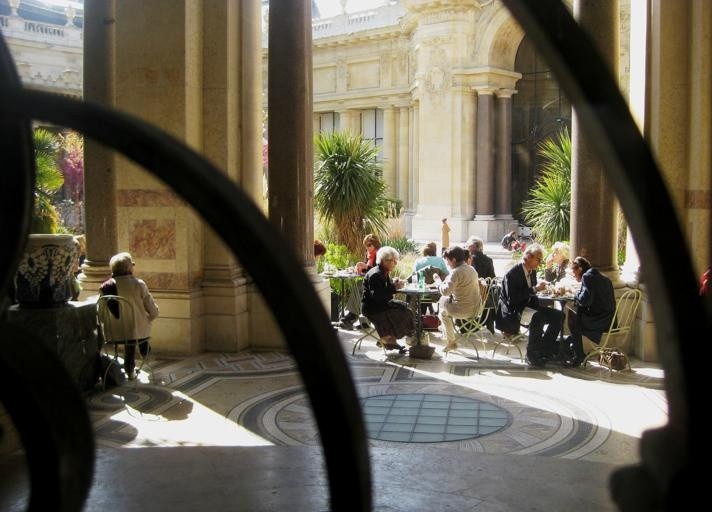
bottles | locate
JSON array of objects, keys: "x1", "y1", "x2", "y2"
[
  {"x1": 419, "y1": 272, "x2": 424, "y2": 288},
  {"x1": 412, "y1": 272, "x2": 417, "y2": 285}
]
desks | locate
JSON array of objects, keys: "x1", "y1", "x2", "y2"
[
  {"x1": 534, "y1": 286, "x2": 578, "y2": 367},
  {"x1": 322, "y1": 271, "x2": 363, "y2": 326},
  {"x1": 392, "y1": 284, "x2": 441, "y2": 361}
]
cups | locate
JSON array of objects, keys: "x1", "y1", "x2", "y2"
[{"x1": 560, "y1": 286, "x2": 565, "y2": 296}]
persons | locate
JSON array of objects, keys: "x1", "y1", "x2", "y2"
[
  {"x1": 97, "y1": 250, "x2": 159, "y2": 373},
  {"x1": 558, "y1": 254, "x2": 620, "y2": 370},
  {"x1": 312, "y1": 215, "x2": 571, "y2": 366}
]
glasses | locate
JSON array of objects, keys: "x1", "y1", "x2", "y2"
[{"x1": 367, "y1": 243, "x2": 371, "y2": 248}]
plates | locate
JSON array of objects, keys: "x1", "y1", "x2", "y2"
[
  {"x1": 430, "y1": 287, "x2": 436, "y2": 289},
  {"x1": 324, "y1": 273, "x2": 334, "y2": 275}
]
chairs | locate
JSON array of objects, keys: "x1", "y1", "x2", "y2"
[
  {"x1": 579, "y1": 289, "x2": 642, "y2": 376},
  {"x1": 445, "y1": 276, "x2": 529, "y2": 363},
  {"x1": 95, "y1": 295, "x2": 153, "y2": 392},
  {"x1": 349, "y1": 276, "x2": 388, "y2": 356}
]
